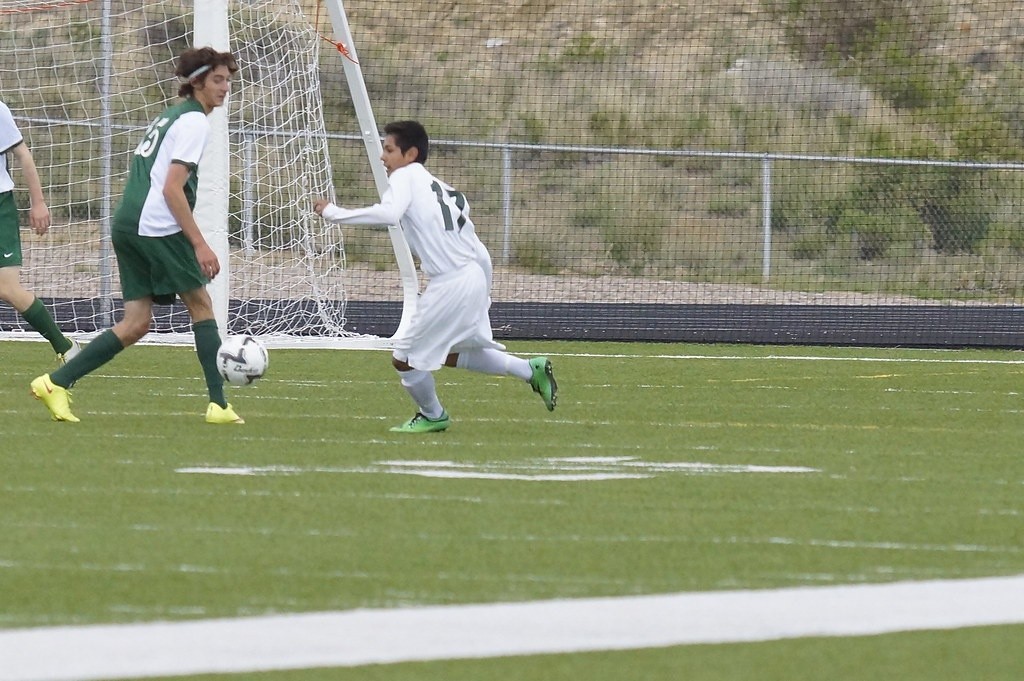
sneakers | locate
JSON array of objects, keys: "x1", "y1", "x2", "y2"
[
  {"x1": 205, "y1": 401, "x2": 245, "y2": 424},
  {"x1": 57, "y1": 336, "x2": 82, "y2": 387},
  {"x1": 526, "y1": 356, "x2": 558, "y2": 411},
  {"x1": 29, "y1": 372, "x2": 80, "y2": 423},
  {"x1": 388, "y1": 406, "x2": 450, "y2": 434}
]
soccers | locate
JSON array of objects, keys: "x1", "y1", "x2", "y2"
[{"x1": 215, "y1": 334, "x2": 270, "y2": 387}]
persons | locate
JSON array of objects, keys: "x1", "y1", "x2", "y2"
[
  {"x1": 31, "y1": 48, "x2": 245, "y2": 424},
  {"x1": 313, "y1": 120, "x2": 558, "y2": 433},
  {"x1": 0, "y1": 102, "x2": 81, "y2": 365}
]
